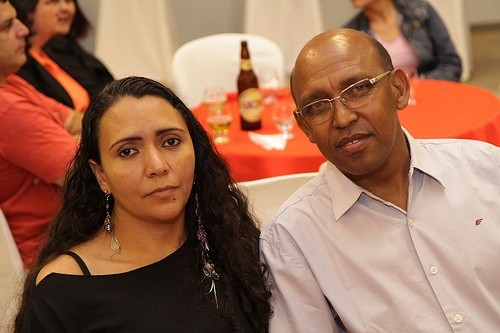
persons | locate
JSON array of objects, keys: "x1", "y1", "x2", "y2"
[
  {"x1": 259, "y1": 28, "x2": 500, "y2": 333},
  {"x1": 8, "y1": 0, "x2": 115, "y2": 111},
  {"x1": 11, "y1": 76, "x2": 275, "y2": 333},
  {"x1": 340, "y1": 0, "x2": 463, "y2": 82},
  {"x1": 0, "y1": 0, "x2": 87, "y2": 269}
]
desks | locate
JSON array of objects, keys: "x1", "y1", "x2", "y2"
[{"x1": 190, "y1": 78, "x2": 500, "y2": 182}]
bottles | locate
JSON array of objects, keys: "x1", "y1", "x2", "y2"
[{"x1": 237, "y1": 41, "x2": 262, "y2": 131}]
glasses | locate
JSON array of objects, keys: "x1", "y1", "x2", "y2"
[{"x1": 294, "y1": 71, "x2": 393, "y2": 126}]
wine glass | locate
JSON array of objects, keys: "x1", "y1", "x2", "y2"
[
  {"x1": 204, "y1": 85, "x2": 232, "y2": 144},
  {"x1": 395, "y1": 65, "x2": 419, "y2": 105},
  {"x1": 271, "y1": 99, "x2": 296, "y2": 140}
]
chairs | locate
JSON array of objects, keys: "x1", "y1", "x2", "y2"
[
  {"x1": 0, "y1": 209, "x2": 26, "y2": 333},
  {"x1": 236, "y1": 172, "x2": 319, "y2": 229},
  {"x1": 172, "y1": 31, "x2": 284, "y2": 110}
]
